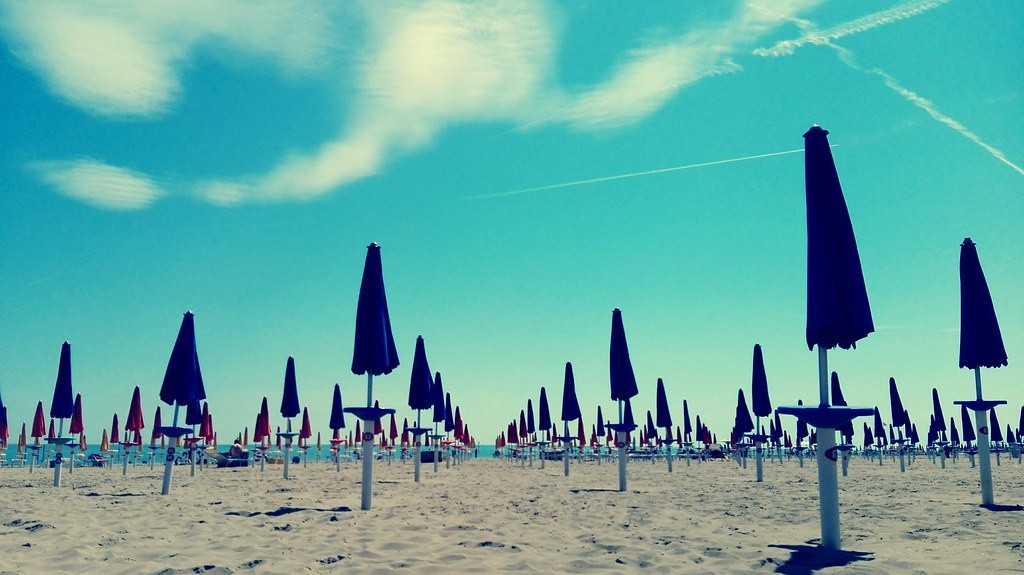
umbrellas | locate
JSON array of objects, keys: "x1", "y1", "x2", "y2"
[
  {"x1": 17, "y1": 418, "x2": 56, "y2": 453},
  {"x1": 239, "y1": 383, "x2": 417, "y2": 448},
  {"x1": 158, "y1": 310, "x2": 207, "y2": 426},
  {"x1": 110, "y1": 414, "x2": 120, "y2": 450},
  {"x1": 69, "y1": 393, "x2": 82, "y2": 439},
  {"x1": 28, "y1": 399, "x2": 47, "y2": 445},
  {"x1": 749, "y1": 342, "x2": 773, "y2": 435},
  {"x1": 607, "y1": 306, "x2": 641, "y2": 424},
  {"x1": 99, "y1": 429, "x2": 109, "y2": 453},
  {"x1": 278, "y1": 354, "x2": 300, "y2": 433},
  {"x1": 407, "y1": 334, "x2": 476, "y2": 451},
  {"x1": 0, "y1": 395, "x2": 9, "y2": 451},
  {"x1": 494, "y1": 361, "x2": 1024, "y2": 447},
  {"x1": 77, "y1": 430, "x2": 87, "y2": 452},
  {"x1": 49, "y1": 341, "x2": 74, "y2": 437},
  {"x1": 349, "y1": 241, "x2": 399, "y2": 408},
  {"x1": 802, "y1": 123, "x2": 877, "y2": 406},
  {"x1": 123, "y1": 385, "x2": 144, "y2": 443},
  {"x1": 122, "y1": 400, "x2": 217, "y2": 451},
  {"x1": 956, "y1": 238, "x2": 1009, "y2": 399}
]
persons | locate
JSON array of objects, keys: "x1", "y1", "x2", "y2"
[{"x1": 228, "y1": 438, "x2": 243, "y2": 467}]
